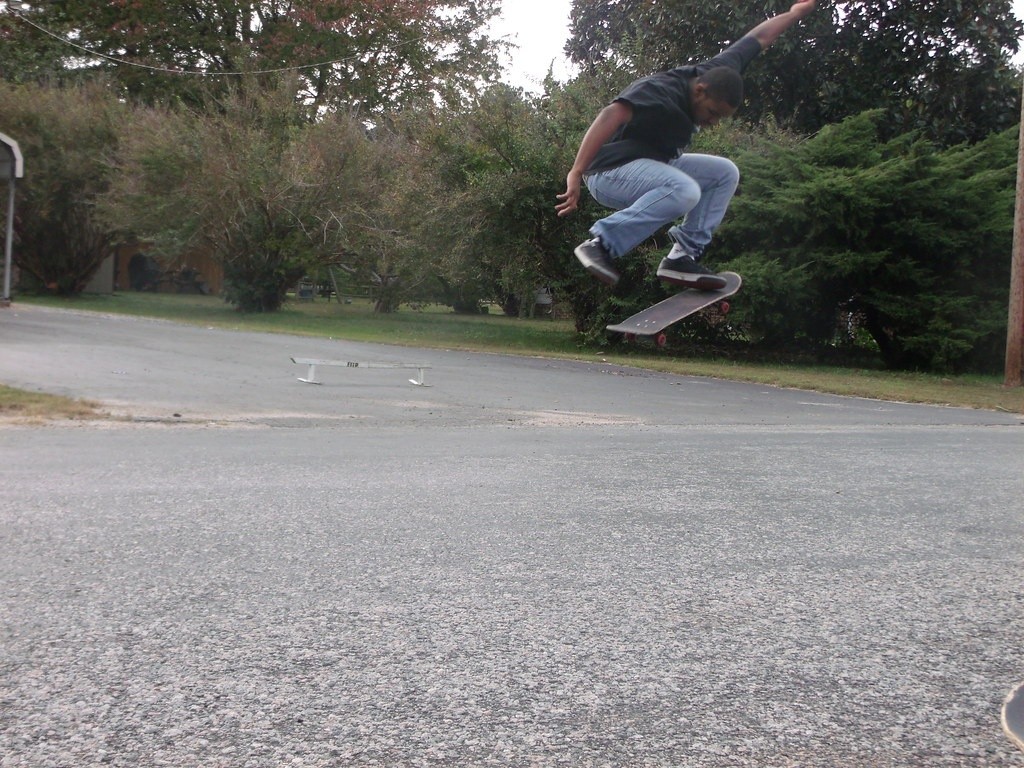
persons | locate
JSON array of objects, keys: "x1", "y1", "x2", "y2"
[{"x1": 553, "y1": 0, "x2": 818, "y2": 291}]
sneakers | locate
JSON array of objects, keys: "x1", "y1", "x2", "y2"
[
  {"x1": 657, "y1": 255, "x2": 728, "y2": 290},
  {"x1": 574, "y1": 239, "x2": 620, "y2": 283}
]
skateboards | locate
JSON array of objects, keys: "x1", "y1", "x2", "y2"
[{"x1": 605, "y1": 271, "x2": 742, "y2": 347}]
